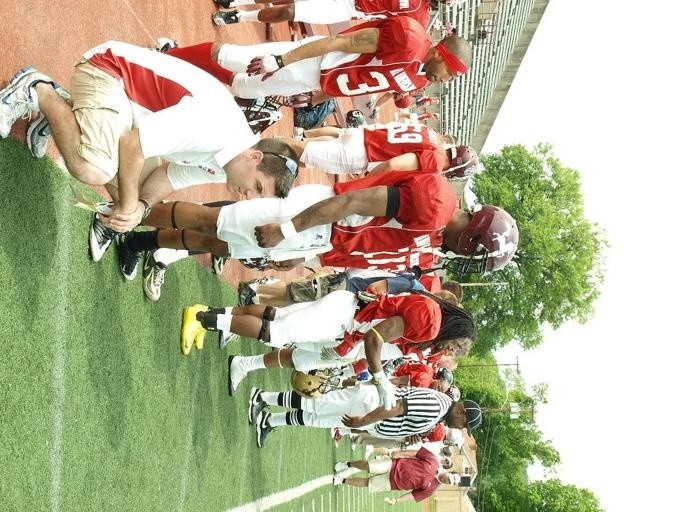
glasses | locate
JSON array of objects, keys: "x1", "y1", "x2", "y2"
[{"x1": 262, "y1": 150, "x2": 298, "y2": 178}]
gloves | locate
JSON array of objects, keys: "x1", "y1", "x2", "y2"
[
  {"x1": 375, "y1": 372, "x2": 397, "y2": 411},
  {"x1": 247, "y1": 54, "x2": 279, "y2": 81}
]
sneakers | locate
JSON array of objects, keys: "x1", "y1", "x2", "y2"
[
  {"x1": 117, "y1": 232, "x2": 140, "y2": 280},
  {"x1": 181, "y1": 307, "x2": 201, "y2": 355},
  {"x1": 366, "y1": 446, "x2": 373, "y2": 459},
  {"x1": 196, "y1": 305, "x2": 207, "y2": 349},
  {"x1": 213, "y1": 0, "x2": 234, "y2": 8},
  {"x1": 351, "y1": 435, "x2": 358, "y2": 451},
  {"x1": 334, "y1": 478, "x2": 343, "y2": 486},
  {"x1": 154, "y1": 39, "x2": 176, "y2": 53},
  {"x1": 336, "y1": 432, "x2": 342, "y2": 448},
  {"x1": 89, "y1": 202, "x2": 115, "y2": 262},
  {"x1": 238, "y1": 284, "x2": 255, "y2": 308},
  {"x1": 213, "y1": 10, "x2": 239, "y2": 25},
  {"x1": 331, "y1": 428, "x2": 337, "y2": 438},
  {"x1": 213, "y1": 255, "x2": 224, "y2": 275},
  {"x1": 143, "y1": 249, "x2": 167, "y2": 302},
  {"x1": 335, "y1": 461, "x2": 348, "y2": 473},
  {"x1": 257, "y1": 411, "x2": 272, "y2": 446},
  {"x1": 229, "y1": 356, "x2": 246, "y2": 397},
  {"x1": 27, "y1": 83, "x2": 71, "y2": 158},
  {"x1": 220, "y1": 331, "x2": 234, "y2": 348},
  {"x1": 0, "y1": 67, "x2": 53, "y2": 138},
  {"x1": 249, "y1": 387, "x2": 266, "y2": 423}
]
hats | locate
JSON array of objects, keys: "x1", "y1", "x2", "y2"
[
  {"x1": 450, "y1": 474, "x2": 460, "y2": 486},
  {"x1": 464, "y1": 399, "x2": 482, "y2": 437}
]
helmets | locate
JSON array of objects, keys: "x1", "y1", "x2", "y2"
[
  {"x1": 445, "y1": 147, "x2": 477, "y2": 181},
  {"x1": 434, "y1": 368, "x2": 452, "y2": 384},
  {"x1": 347, "y1": 110, "x2": 365, "y2": 128},
  {"x1": 447, "y1": 206, "x2": 518, "y2": 273},
  {"x1": 235, "y1": 96, "x2": 282, "y2": 135},
  {"x1": 292, "y1": 371, "x2": 327, "y2": 399},
  {"x1": 447, "y1": 385, "x2": 460, "y2": 402}
]
